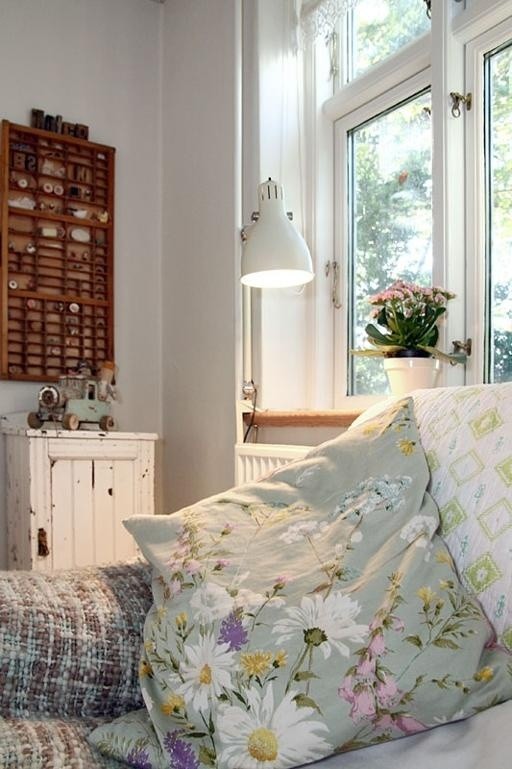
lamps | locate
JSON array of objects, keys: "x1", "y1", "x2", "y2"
[{"x1": 238, "y1": 177, "x2": 315, "y2": 290}]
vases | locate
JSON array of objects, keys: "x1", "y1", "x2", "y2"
[{"x1": 382, "y1": 351, "x2": 439, "y2": 396}]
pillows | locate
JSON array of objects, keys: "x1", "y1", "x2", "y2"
[{"x1": 85, "y1": 394, "x2": 510, "y2": 769}]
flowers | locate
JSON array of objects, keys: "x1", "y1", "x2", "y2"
[{"x1": 348, "y1": 278, "x2": 468, "y2": 366}]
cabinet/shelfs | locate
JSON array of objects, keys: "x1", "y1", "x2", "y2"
[
  {"x1": 0, "y1": 428, "x2": 158, "y2": 572},
  {"x1": 0, "y1": 119, "x2": 115, "y2": 384}
]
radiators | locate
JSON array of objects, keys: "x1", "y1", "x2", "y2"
[{"x1": 234, "y1": 443, "x2": 315, "y2": 487}]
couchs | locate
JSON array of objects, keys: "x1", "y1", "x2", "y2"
[{"x1": 0, "y1": 380, "x2": 510, "y2": 769}]
